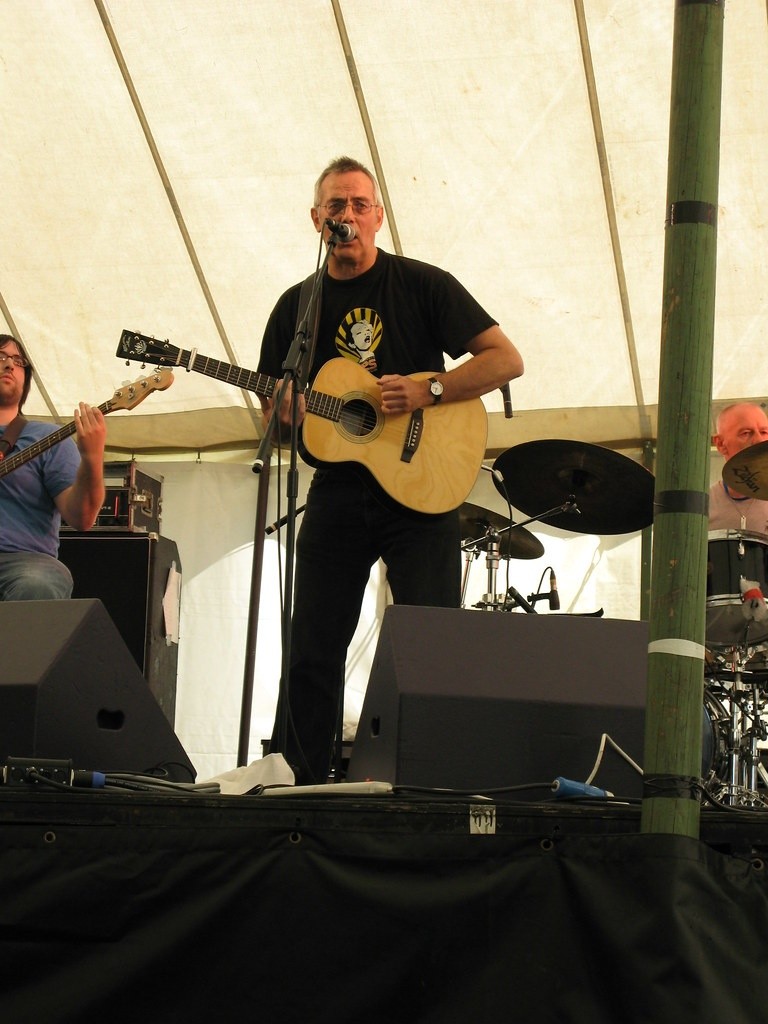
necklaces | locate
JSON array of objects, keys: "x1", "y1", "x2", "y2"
[{"x1": 719, "y1": 483, "x2": 755, "y2": 529}]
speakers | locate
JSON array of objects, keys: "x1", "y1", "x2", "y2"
[
  {"x1": 56, "y1": 530, "x2": 182, "y2": 731},
  {"x1": 0, "y1": 598, "x2": 198, "y2": 784},
  {"x1": 345, "y1": 604, "x2": 713, "y2": 803}
]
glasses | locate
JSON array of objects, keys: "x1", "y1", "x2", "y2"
[
  {"x1": 0, "y1": 350, "x2": 31, "y2": 368},
  {"x1": 315, "y1": 198, "x2": 378, "y2": 214}
]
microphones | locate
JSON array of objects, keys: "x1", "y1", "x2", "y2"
[
  {"x1": 325, "y1": 217, "x2": 356, "y2": 242},
  {"x1": 549, "y1": 571, "x2": 560, "y2": 610}
]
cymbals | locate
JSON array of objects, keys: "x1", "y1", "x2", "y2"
[
  {"x1": 488, "y1": 435, "x2": 661, "y2": 538},
  {"x1": 718, "y1": 439, "x2": 768, "y2": 507},
  {"x1": 452, "y1": 498, "x2": 546, "y2": 559}
]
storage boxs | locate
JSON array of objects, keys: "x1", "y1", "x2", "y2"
[{"x1": 59, "y1": 461, "x2": 163, "y2": 533}]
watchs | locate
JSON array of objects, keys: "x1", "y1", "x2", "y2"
[{"x1": 428, "y1": 375, "x2": 444, "y2": 406}]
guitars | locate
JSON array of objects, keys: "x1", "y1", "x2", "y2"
[
  {"x1": 0, "y1": 358, "x2": 176, "y2": 483},
  {"x1": 111, "y1": 323, "x2": 495, "y2": 513}
]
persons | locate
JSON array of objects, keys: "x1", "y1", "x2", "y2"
[
  {"x1": 0, "y1": 335, "x2": 107, "y2": 602},
  {"x1": 701, "y1": 402, "x2": 768, "y2": 534},
  {"x1": 255, "y1": 157, "x2": 525, "y2": 787}
]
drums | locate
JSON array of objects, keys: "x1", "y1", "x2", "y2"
[
  {"x1": 695, "y1": 682, "x2": 736, "y2": 784},
  {"x1": 701, "y1": 526, "x2": 768, "y2": 657}
]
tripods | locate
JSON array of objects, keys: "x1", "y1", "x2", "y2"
[{"x1": 700, "y1": 644, "x2": 768, "y2": 808}]
